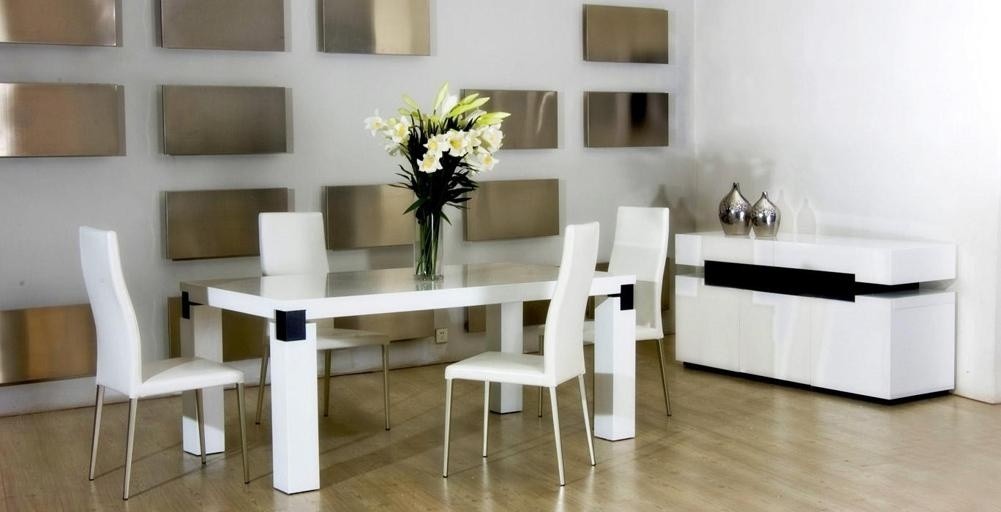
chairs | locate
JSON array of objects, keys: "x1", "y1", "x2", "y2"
[
  {"x1": 441, "y1": 220, "x2": 602, "y2": 488},
  {"x1": 254, "y1": 208, "x2": 391, "y2": 432},
  {"x1": 536, "y1": 204, "x2": 673, "y2": 433},
  {"x1": 76, "y1": 224, "x2": 251, "y2": 501}
]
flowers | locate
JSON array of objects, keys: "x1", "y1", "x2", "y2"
[{"x1": 361, "y1": 80, "x2": 512, "y2": 279}]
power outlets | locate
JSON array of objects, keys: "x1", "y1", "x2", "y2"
[{"x1": 435, "y1": 326, "x2": 450, "y2": 347}]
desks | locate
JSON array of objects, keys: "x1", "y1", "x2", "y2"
[{"x1": 178, "y1": 258, "x2": 637, "y2": 458}]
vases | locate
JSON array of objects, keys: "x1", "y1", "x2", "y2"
[{"x1": 413, "y1": 205, "x2": 444, "y2": 284}]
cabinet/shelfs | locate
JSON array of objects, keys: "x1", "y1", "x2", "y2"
[{"x1": 674, "y1": 227, "x2": 959, "y2": 406}]
